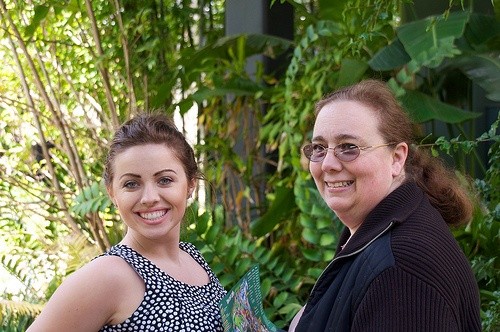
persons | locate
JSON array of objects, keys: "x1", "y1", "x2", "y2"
[
  {"x1": 287, "y1": 79, "x2": 482, "y2": 332},
  {"x1": 26, "y1": 112, "x2": 227, "y2": 332}
]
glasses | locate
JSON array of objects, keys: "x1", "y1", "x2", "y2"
[{"x1": 302, "y1": 141, "x2": 396, "y2": 164}]
